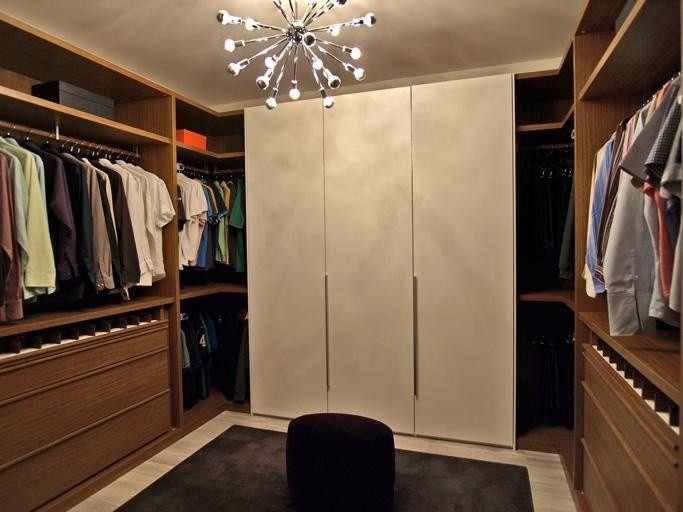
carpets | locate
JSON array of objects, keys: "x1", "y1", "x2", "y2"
[{"x1": 109, "y1": 424, "x2": 535, "y2": 512}]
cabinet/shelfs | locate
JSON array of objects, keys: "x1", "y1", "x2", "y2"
[
  {"x1": 0, "y1": 9, "x2": 250, "y2": 512},
  {"x1": 516, "y1": 0, "x2": 683, "y2": 511},
  {"x1": 244, "y1": 72, "x2": 517, "y2": 449}
]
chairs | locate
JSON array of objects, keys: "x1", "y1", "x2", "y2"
[{"x1": 286, "y1": 413, "x2": 395, "y2": 512}]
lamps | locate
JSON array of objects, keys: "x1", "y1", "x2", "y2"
[{"x1": 216, "y1": 0, "x2": 377, "y2": 110}]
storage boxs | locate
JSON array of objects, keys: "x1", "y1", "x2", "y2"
[
  {"x1": 176, "y1": 129, "x2": 207, "y2": 150},
  {"x1": 31, "y1": 79, "x2": 114, "y2": 121}
]
